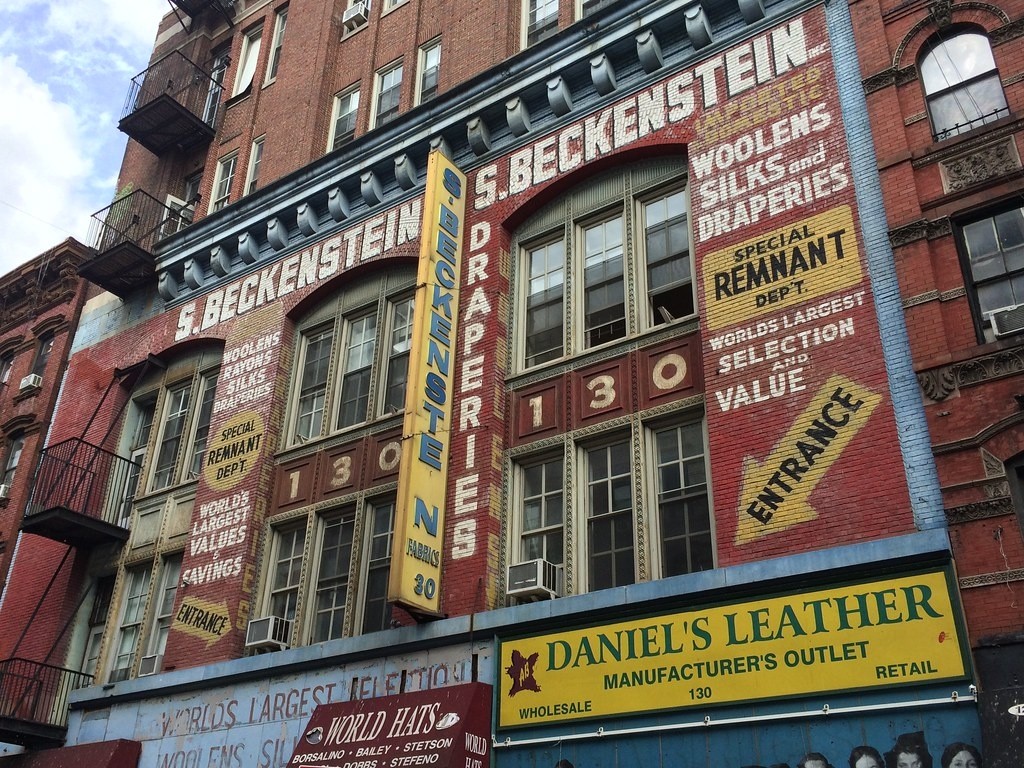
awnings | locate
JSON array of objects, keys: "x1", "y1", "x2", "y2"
[
  {"x1": 0, "y1": 738, "x2": 141, "y2": 768},
  {"x1": 286, "y1": 682, "x2": 493, "y2": 768}
]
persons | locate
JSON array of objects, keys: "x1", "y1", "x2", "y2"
[{"x1": 799, "y1": 731, "x2": 982, "y2": 768}]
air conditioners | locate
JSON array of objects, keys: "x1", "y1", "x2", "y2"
[
  {"x1": 0, "y1": 484, "x2": 9, "y2": 500},
  {"x1": 506, "y1": 557, "x2": 562, "y2": 604},
  {"x1": 19, "y1": 374, "x2": 42, "y2": 391},
  {"x1": 245, "y1": 616, "x2": 294, "y2": 649},
  {"x1": 342, "y1": 2, "x2": 369, "y2": 30},
  {"x1": 138, "y1": 654, "x2": 163, "y2": 677},
  {"x1": 989, "y1": 303, "x2": 1024, "y2": 340}
]
